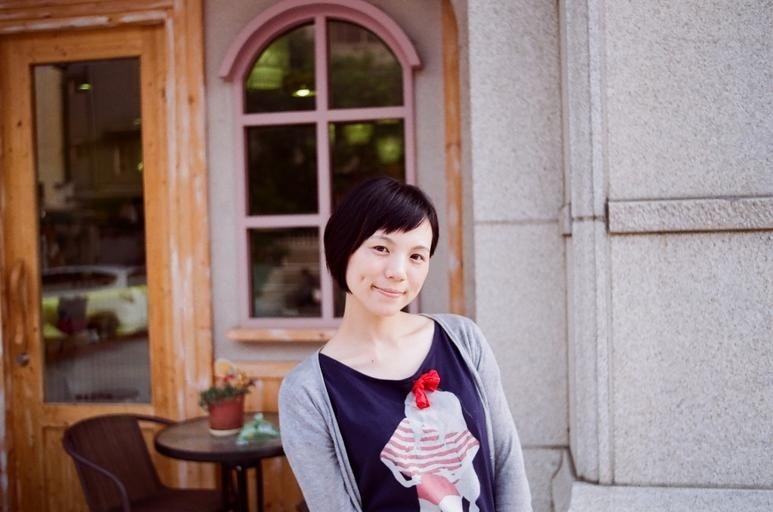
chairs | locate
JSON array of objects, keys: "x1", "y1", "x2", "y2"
[{"x1": 60, "y1": 413, "x2": 245, "y2": 511}]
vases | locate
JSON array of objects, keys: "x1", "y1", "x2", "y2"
[{"x1": 204, "y1": 395, "x2": 246, "y2": 437}]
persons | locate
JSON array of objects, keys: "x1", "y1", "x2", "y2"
[{"x1": 278, "y1": 176, "x2": 533, "y2": 512}]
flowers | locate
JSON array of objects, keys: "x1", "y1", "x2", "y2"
[{"x1": 194, "y1": 357, "x2": 259, "y2": 410}]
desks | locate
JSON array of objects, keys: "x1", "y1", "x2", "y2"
[{"x1": 154, "y1": 410, "x2": 288, "y2": 511}]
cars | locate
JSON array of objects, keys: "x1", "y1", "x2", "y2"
[{"x1": 44, "y1": 263, "x2": 148, "y2": 339}]
